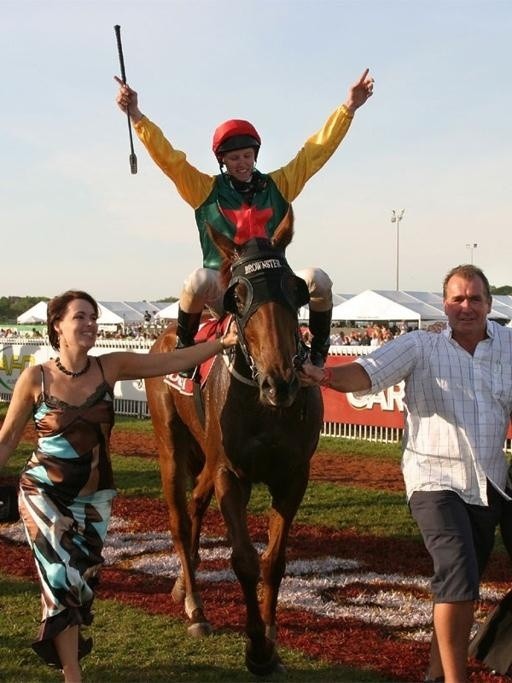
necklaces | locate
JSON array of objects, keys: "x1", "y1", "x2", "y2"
[{"x1": 50, "y1": 355, "x2": 90, "y2": 379}]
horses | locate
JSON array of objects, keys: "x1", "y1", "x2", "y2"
[{"x1": 144, "y1": 203, "x2": 325, "y2": 677}]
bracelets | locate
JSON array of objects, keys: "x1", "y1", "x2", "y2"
[
  {"x1": 217, "y1": 336, "x2": 223, "y2": 353},
  {"x1": 321, "y1": 364, "x2": 335, "y2": 389}
]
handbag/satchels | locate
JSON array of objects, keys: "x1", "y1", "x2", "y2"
[{"x1": 0, "y1": 484, "x2": 20, "y2": 524}]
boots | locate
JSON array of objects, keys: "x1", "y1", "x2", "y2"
[
  {"x1": 174, "y1": 304, "x2": 202, "y2": 378},
  {"x1": 308, "y1": 307, "x2": 333, "y2": 369}
]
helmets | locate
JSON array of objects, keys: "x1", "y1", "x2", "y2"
[{"x1": 212, "y1": 118, "x2": 261, "y2": 160}]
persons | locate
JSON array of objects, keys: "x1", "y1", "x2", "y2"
[
  {"x1": 297, "y1": 323, "x2": 311, "y2": 348},
  {"x1": 97, "y1": 309, "x2": 169, "y2": 341},
  {"x1": 0, "y1": 327, "x2": 49, "y2": 339},
  {"x1": 293, "y1": 264, "x2": 512, "y2": 683},
  {"x1": 330, "y1": 321, "x2": 419, "y2": 346},
  {"x1": 0, "y1": 291, "x2": 243, "y2": 683},
  {"x1": 111, "y1": 67, "x2": 375, "y2": 396}
]
